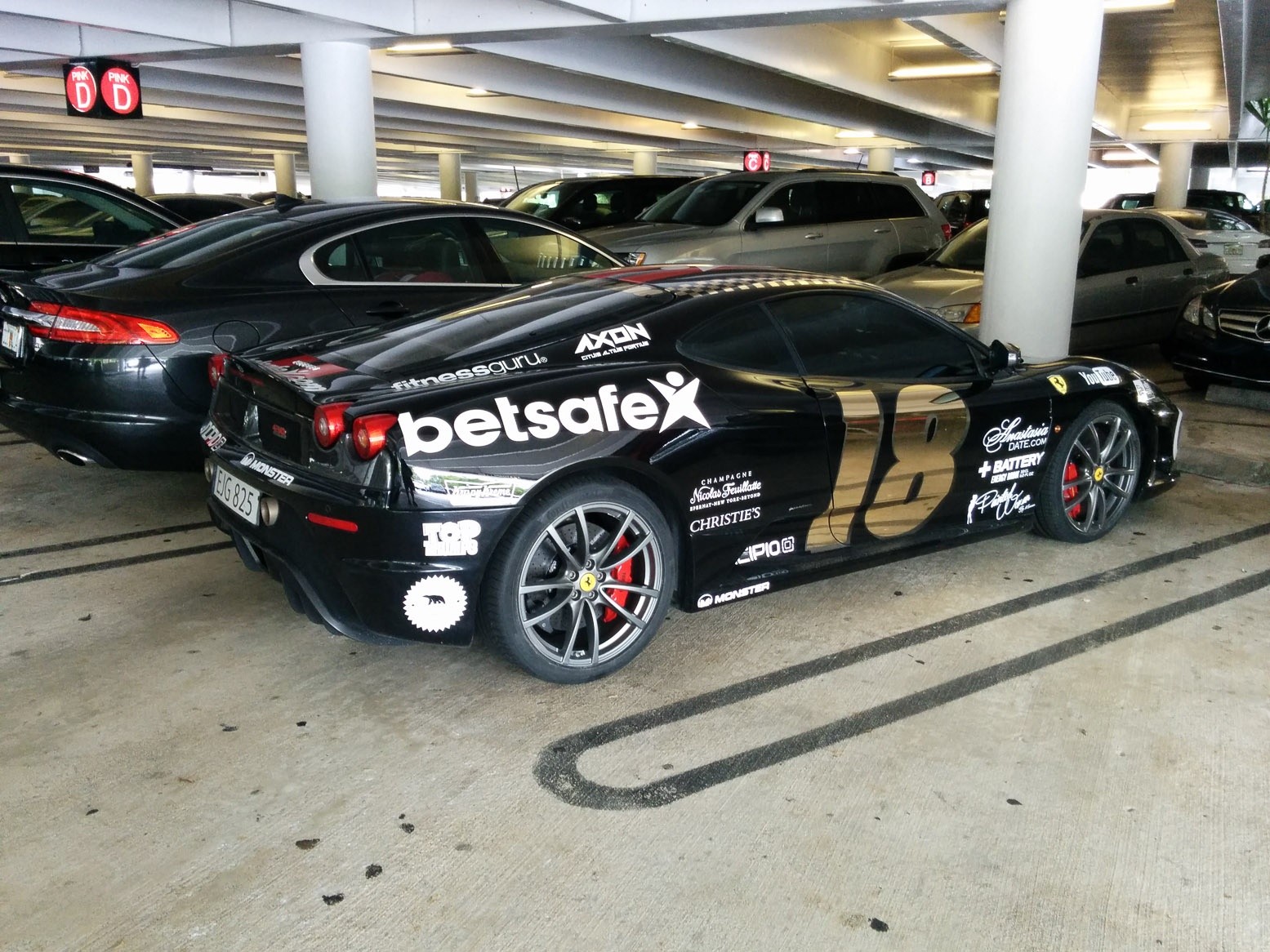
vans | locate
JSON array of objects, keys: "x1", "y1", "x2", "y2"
[{"x1": 490, "y1": 176, "x2": 703, "y2": 280}]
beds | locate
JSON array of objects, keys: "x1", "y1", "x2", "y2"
[{"x1": 369, "y1": 233, "x2": 465, "y2": 281}]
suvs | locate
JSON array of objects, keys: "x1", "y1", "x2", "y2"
[{"x1": 538, "y1": 167, "x2": 951, "y2": 286}]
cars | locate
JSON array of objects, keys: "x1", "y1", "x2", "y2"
[
  {"x1": 1, "y1": 163, "x2": 265, "y2": 284},
  {"x1": 1173, "y1": 251, "x2": 1270, "y2": 400},
  {"x1": 864, "y1": 210, "x2": 1234, "y2": 354},
  {"x1": 0, "y1": 192, "x2": 642, "y2": 471},
  {"x1": 936, "y1": 189, "x2": 990, "y2": 235},
  {"x1": 1105, "y1": 187, "x2": 1270, "y2": 274}
]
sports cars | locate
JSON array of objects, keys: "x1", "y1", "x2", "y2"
[{"x1": 199, "y1": 264, "x2": 1185, "y2": 684}]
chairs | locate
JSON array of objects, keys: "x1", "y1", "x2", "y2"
[
  {"x1": 578, "y1": 191, "x2": 654, "y2": 222},
  {"x1": 1090, "y1": 238, "x2": 1174, "y2": 263}
]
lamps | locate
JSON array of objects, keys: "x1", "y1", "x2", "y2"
[{"x1": 887, "y1": 40, "x2": 996, "y2": 82}]
